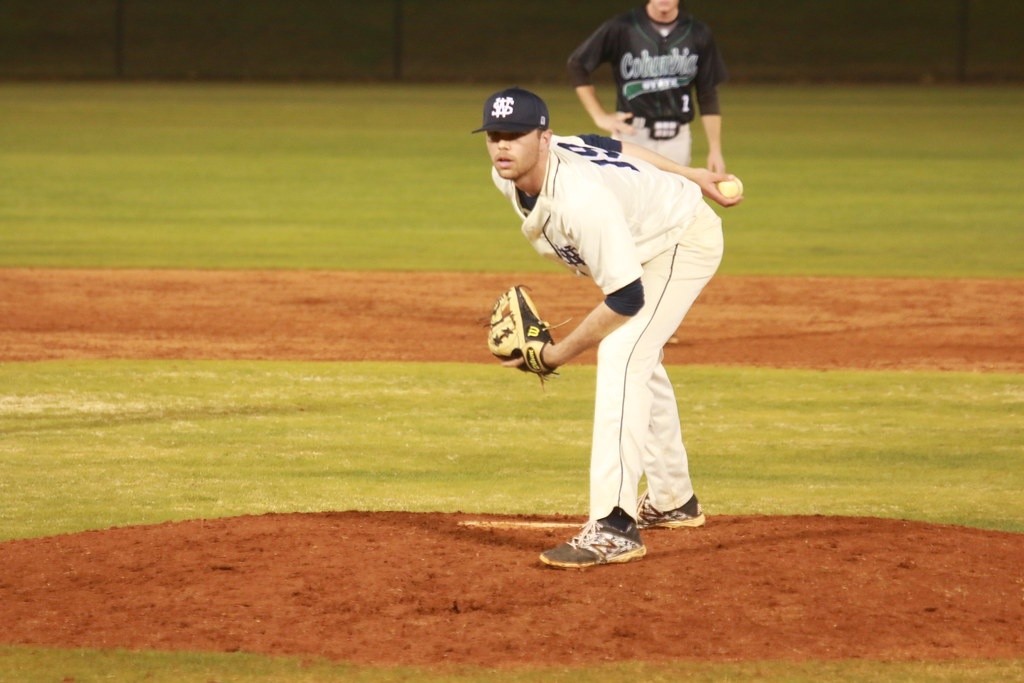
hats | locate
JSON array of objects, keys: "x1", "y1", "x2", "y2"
[{"x1": 471, "y1": 87, "x2": 550, "y2": 133}]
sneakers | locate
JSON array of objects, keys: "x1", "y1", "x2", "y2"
[
  {"x1": 540, "y1": 522, "x2": 647, "y2": 569},
  {"x1": 635, "y1": 492, "x2": 705, "y2": 530}
]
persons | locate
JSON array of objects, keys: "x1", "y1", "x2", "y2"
[
  {"x1": 567, "y1": 1, "x2": 728, "y2": 175},
  {"x1": 472, "y1": 87, "x2": 745, "y2": 567}
]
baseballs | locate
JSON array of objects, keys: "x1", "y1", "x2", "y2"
[{"x1": 716, "y1": 175, "x2": 745, "y2": 200}]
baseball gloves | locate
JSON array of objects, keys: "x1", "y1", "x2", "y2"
[{"x1": 482, "y1": 284, "x2": 558, "y2": 382}]
